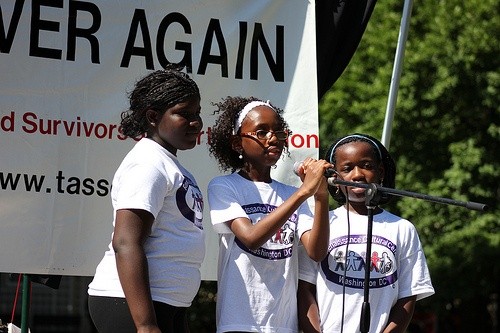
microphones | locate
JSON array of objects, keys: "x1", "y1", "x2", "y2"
[{"x1": 293, "y1": 161, "x2": 335, "y2": 178}]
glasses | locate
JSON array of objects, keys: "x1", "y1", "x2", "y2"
[{"x1": 235, "y1": 129, "x2": 289, "y2": 141}]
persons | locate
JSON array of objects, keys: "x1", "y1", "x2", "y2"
[
  {"x1": 297, "y1": 133, "x2": 435, "y2": 333},
  {"x1": 207, "y1": 96, "x2": 335, "y2": 333},
  {"x1": 87, "y1": 69, "x2": 206, "y2": 333}
]
